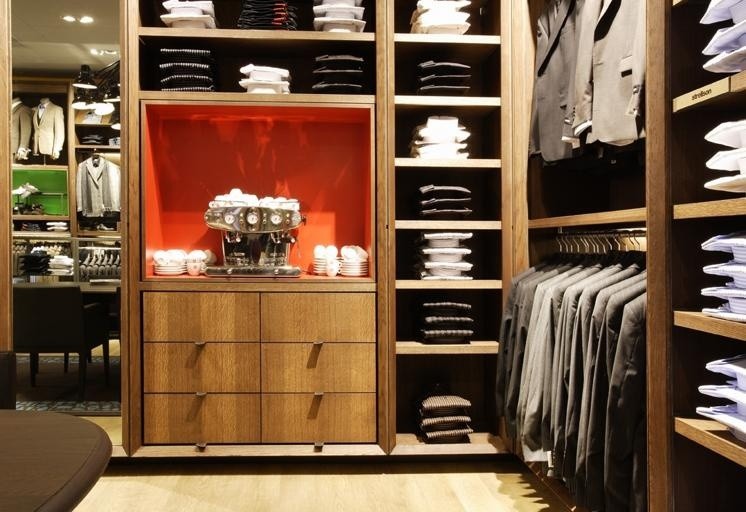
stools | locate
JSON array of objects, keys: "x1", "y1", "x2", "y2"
[{"x1": 0, "y1": 407, "x2": 113, "y2": 512}]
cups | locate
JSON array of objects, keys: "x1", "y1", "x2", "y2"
[
  {"x1": 154, "y1": 249, "x2": 186, "y2": 264},
  {"x1": 312, "y1": 243, "x2": 370, "y2": 261},
  {"x1": 186, "y1": 249, "x2": 217, "y2": 276},
  {"x1": 326, "y1": 259, "x2": 341, "y2": 278},
  {"x1": 207, "y1": 188, "x2": 300, "y2": 210}
]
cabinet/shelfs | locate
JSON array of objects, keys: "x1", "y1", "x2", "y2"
[
  {"x1": 389, "y1": 2, "x2": 511, "y2": 453},
  {"x1": 122, "y1": 2, "x2": 388, "y2": 455},
  {"x1": 512, "y1": 0, "x2": 746, "y2": 511},
  {"x1": 10, "y1": 73, "x2": 120, "y2": 292}
]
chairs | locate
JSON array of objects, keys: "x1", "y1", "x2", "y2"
[{"x1": 9, "y1": 281, "x2": 109, "y2": 395}]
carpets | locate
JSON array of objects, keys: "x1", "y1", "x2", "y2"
[{"x1": 12, "y1": 354, "x2": 120, "y2": 419}]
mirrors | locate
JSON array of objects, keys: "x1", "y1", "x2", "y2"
[{"x1": 5, "y1": 0, "x2": 125, "y2": 447}]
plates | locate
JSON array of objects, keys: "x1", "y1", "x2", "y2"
[
  {"x1": 340, "y1": 260, "x2": 367, "y2": 277},
  {"x1": 154, "y1": 265, "x2": 187, "y2": 276},
  {"x1": 312, "y1": 257, "x2": 327, "y2": 276}
]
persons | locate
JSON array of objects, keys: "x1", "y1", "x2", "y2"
[
  {"x1": 10, "y1": 97, "x2": 33, "y2": 165},
  {"x1": 31, "y1": 97, "x2": 66, "y2": 162}
]
